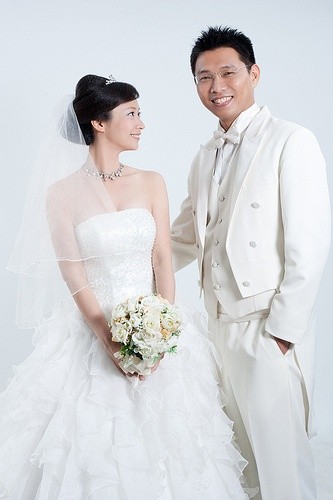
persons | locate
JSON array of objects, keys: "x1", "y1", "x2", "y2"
[
  {"x1": 169, "y1": 26, "x2": 331, "y2": 500},
  {"x1": 0, "y1": 75, "x2": 253, "y2": 499}
]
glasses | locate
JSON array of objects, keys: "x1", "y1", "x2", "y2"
[{"x1": 194, "y1": 63, "x2": 251, "y2": 86}]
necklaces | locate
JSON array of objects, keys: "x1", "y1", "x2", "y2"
[{"x1": 82, "y1": 162, "x2": 124, "y2": 181}]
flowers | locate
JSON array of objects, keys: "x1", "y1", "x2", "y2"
[{"x1": 108, "y1": 294, "x2": 183, "y2": 375}]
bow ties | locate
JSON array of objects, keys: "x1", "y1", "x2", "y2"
[{"x1": 213, "y1": 127, "x2": 240, "y2": 149}]
